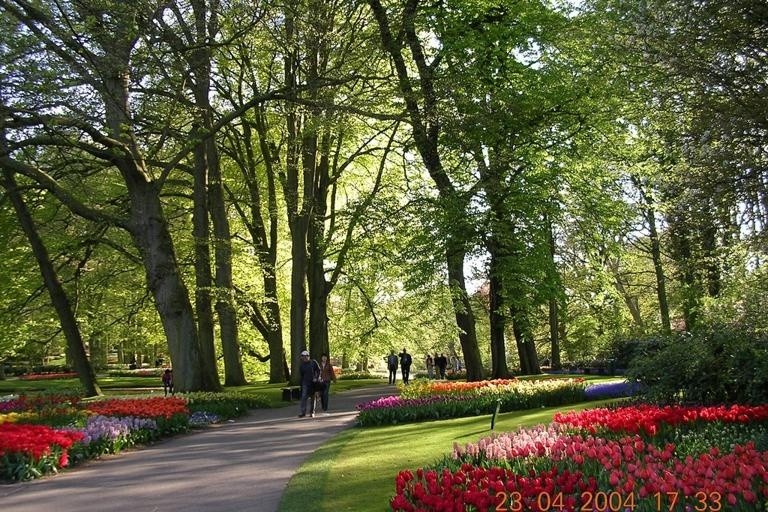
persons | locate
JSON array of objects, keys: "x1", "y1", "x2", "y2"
[
  {"x1": 426, "y1": 353, "x2": 447, "y2": 380},
  {"x1": 388, "y1": 350, "x2": 398, "y2": 384},
  {"x1": 162, "y1": 369, "x2": 174, "y2": 397},
  {"x1": 297, "y1": 351, "x2": 336, "y2": 417},
  {"x1": 450, "y1": 357, "x2": 461, "y2": 372},
  {"x1": 399, "y1": 348, "x2": 412, "y2": 384},
  {"x1": 130, "y1": 361, "x2": 140, "y2": 369}
]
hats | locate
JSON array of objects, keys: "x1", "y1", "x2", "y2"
[{"x1": 301, "y1": 350, "x2": 311, "y2": 356}]
